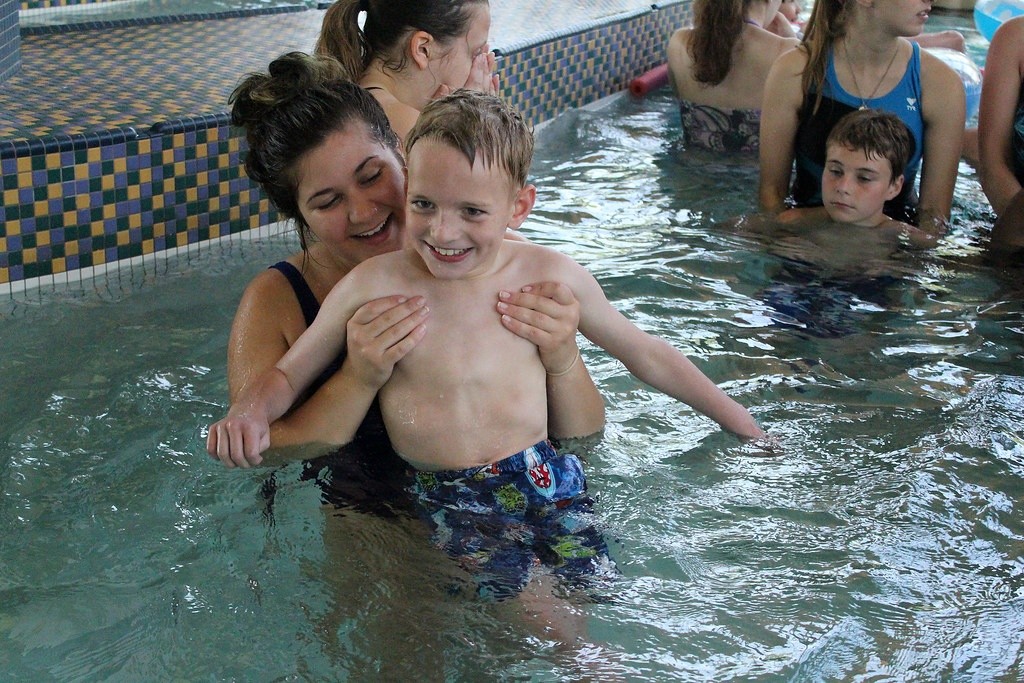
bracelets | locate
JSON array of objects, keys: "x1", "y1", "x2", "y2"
[{"x1": 546, "y1": 350, "x2": 580, "y2": 377}]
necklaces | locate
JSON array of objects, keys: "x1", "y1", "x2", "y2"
[{"x1": 840, "y1": 36, "x2": 901, "y2": 112}]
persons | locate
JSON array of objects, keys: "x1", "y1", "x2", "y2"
[
  {"x1": 228, "y1": 50, "x2": 605, "y2": 517},
  {"x1": 313, "y1": 0, "x2": 501, "y2": 155},
  {"x1": 206, "y1": 92, "x2": 763, "y2": 605},
  {"x1": 665, "y1": 0, "x2": 1024, "y2": 247}
]
